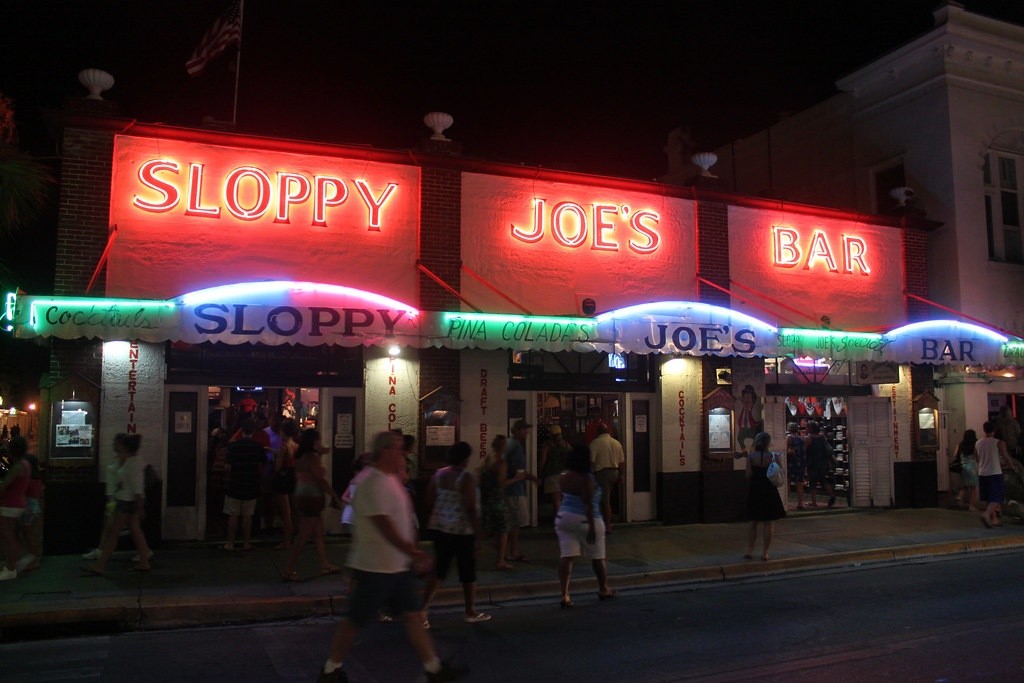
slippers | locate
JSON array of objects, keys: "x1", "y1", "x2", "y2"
[
  {"x1": 464, "y1": 612, "x2": 491, "y2": 623},
  {"x1": 744, "y1": 551, "x2": 772, "y2": 561},
  {"x1": 422, "y1": 621, "x2": 430, "y2": 630},
  {"x1": 79, "y1": 565, "x2": 101, "y2": 576},
  {"x1": 126, "y1": 566, "x2": 152, "y2": 572},
  {"x1": 242, "y1": 545, "x2": 251, "y2": 552},
  {"x1": 217, "y1": 545, "x2": 234, "y2": 552}
]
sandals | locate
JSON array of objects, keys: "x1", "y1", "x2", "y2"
[
  {"x1": 280, "y1": 571, "x2": 306, "y2": 583},
  {"x1": 319, "y1": 565, "x2": 344, "y2": 575}
]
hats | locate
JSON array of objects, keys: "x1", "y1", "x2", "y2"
[
  {"x1": 512, "y1": 419, "x2": 533, "y2": 429},
  {"x1": 551, "y1": 425, "x2": 561, "y2": 435}
]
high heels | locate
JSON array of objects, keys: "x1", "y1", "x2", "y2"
[
  {"x1": 599, "y1": 590, "x2": 616, "y2": 601},
  {"x1": 559, "y1": 599, "x2": 576, "y2": 611}
]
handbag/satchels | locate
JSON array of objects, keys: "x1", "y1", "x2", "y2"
[
  {"x1": 765, "y1": 451, "x2": 784, "y2": 487},
  {"x1": 824, "y1": 440, "x2": 832, "y2": 456},
  {"x1": 951, "y1": 458, "x2": 962, "y2": 473}
]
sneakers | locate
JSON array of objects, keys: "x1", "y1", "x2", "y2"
[{"x1": 0, "y1": 566, "x2": 18, "y2": 581}]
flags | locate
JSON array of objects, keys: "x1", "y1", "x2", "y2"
[{"x1": 184, "y1": 0, "x2": 243, "y2": 79}]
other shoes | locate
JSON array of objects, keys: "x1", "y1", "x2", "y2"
[
  {"x1": 316, "y1": 665, "x2": 349, "y2": 683},
  {"x1": 422, "y1": 657, "x2": 471, "y2": 683},
  {"x1": 82, "y1": 549, "x2": 102, "y2": 560},
  {"x1": 795, "y1": 495, "x2": 837, "y2": 509},
  {"x1": 371, "y1": 616, "x2": 392, "y2": 622},
  {"x1": 495, "y1": 561, "x2": 515, "y2": 569},
  {"x1": 27, "y1": 562, "x2": 40, "y2": 570},
  {"x1": 505, "y1": 551, "x2": 529, "y2": 561},
  {"x1": 954, "y1": 496, "x2": 1003, "y2": 528},
  {"x1": 130, "y1": 551, "x2": 153, "y2": 562}
]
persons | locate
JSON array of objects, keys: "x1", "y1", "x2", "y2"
[
  {"x1": 418, "y1": 440, "x2": 492, "y2": 630},
  {"x1": 0, "y1": 408, "x2": 625, "y2": 683},
  {"x1": 787, "y1": 419, "x2": 835, "y2": 509},
  {"x1": 341, "y1": 427, "x2": 365, "y2": 581},
  {"x1": 949, "y1": 405, "x2": 1024, "y2": 528},
  {"x1": 555, "y1": 444, "x2": 615, "y2": 610},
  {"x1": 744, "y1": 431, "x2": 782, "y2": 561},
  {"x1": 219, "y1": 421, "x2": 267, "y2": 550}
]
